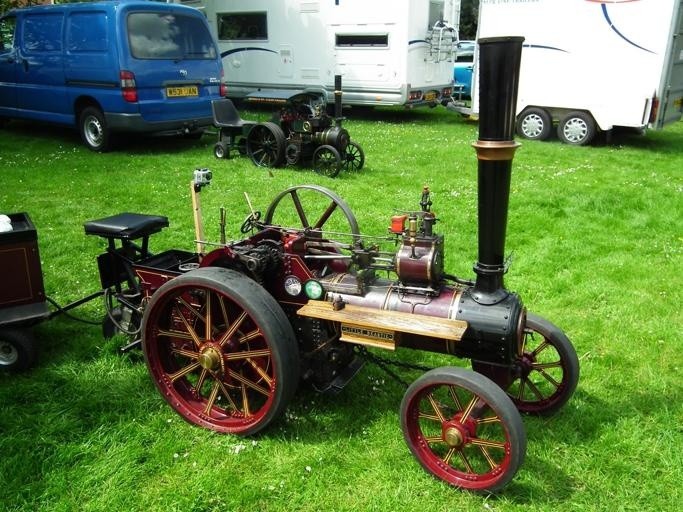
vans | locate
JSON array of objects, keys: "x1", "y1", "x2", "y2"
[{"x1": 0, "y1": 0, "x2": 227, "y2": 150}]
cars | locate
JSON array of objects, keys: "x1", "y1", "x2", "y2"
[{"x1": 454, "y1": 40, "x2": 476, "y2": 98}]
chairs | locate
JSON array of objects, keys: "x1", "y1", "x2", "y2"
[{"x1": 211, "y1": 99, "x2": 258, "y2": 159}]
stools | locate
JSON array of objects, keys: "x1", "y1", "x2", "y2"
[{"x1": 82, "y1": 211, "x2": 169, "y2": 296}]
